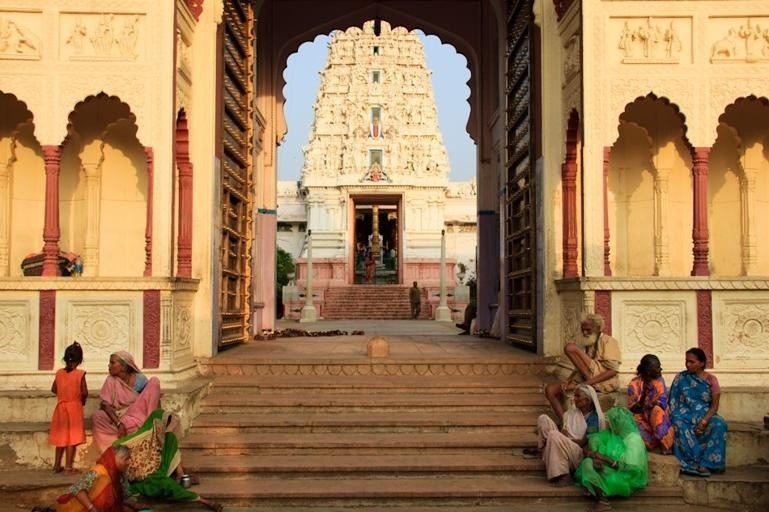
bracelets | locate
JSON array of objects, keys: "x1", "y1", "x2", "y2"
[
  {"x1": 87, "y1": 502, "x2": 94, "y2": 511},
  {"x1": 115, "y1": 420, "x2": 122, "y2": 427}
]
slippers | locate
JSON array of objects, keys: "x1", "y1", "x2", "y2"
[
  {"x1": 686, "y1": 465, "x2": 699, "y2": 475},
  {"x1": 699, "y1": 467, "x2": 711, "y2": 477}
]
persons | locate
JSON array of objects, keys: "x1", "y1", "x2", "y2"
[
  {"x1": 629, "y1": 354, "x2": 675, "y2": 455},
  {"x1": 409, "y1": 281, "x2": 422, "y2": 319},
  {"x1": 91, "y1": 350, "x2": 201, "y2": 485},
  {"x1": 456, "y1": 303, "x2": 477, "y2": 336},
  {"x1": 546, "y1": 314, "x2": 621, "y2": 420},
  {"x1": 574, "y1": 407, "x2": 649, "y2": 511},
  {"x1": 50, "y1": 340, "x2": 88, "y2": 476},
  {"x1": 109, "y1": 408, "x2": 226, "y2": 511},
  {"x1": 43, "y1": 444, "x2": 132, "y2": 512},
  {"x1": 364, "y1": 251, "x2": 376, "y2": 284},
  {"x1": 537, "y1": 385, "x2": 607, "y2": 488},
  {"x1": 669, "y1": 348, "x2": 729, "y2": 476}
]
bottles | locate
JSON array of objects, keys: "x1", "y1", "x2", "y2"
[{"x1": 181, "y1": 475, "x2": 191, "y2": 488}]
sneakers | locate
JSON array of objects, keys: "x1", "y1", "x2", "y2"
[
  {"x1": 456, "y1": 324, "x2": 466, "y2": 331},
  {"x1": 458, "y1": 330, "x2": 470, "y2": 336}
]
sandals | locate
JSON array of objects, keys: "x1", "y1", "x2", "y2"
[
  {"x1": 207, "y1": 501, "x2": 224, "y2": 512},
  {"x1": 175, "y1": 476, "x2": 199, "y2": 486},
  {"x1": 52, "y1": 466, "x2": 64, "y2": 474},
  {"x1": 585, "y1": 500, "x2": 613, "y2": 512},
  {"x1": 64, "y1": 467, "x2": 81, "y2": 476},
  {"x1": 552, "y1": 478, "x2": 575, "y2": 489},
  {"x1": 524, "y1": 450, "x2": 542, "y2": 460}
]
